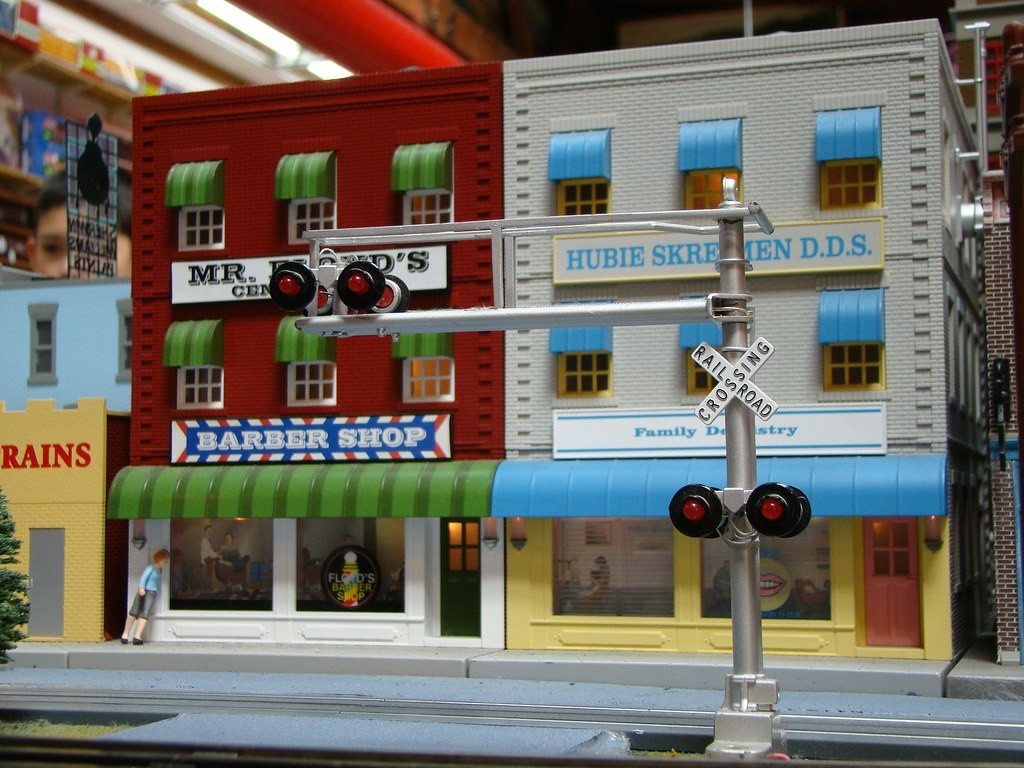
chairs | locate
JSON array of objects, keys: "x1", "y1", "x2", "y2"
[{"x1": 213, "y1": 559, "x2": 240, "y2": 583}]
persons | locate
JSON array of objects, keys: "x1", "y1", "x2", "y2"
[
  {"x1": 27, "y1": 167, "x2": 130, "y2": 277},
  {"x1": 121, "y1": 550, "x2": 170, "y2": 645}
]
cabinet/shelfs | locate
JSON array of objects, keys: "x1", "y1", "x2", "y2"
[{"x1": 0, "y1": 32, "x2": 136, "y2": 269}]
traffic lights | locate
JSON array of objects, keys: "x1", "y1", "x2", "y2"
[
  {"x1": 269, "y1": 264, "x2": 386, "y2": 315},
  {"x1": 672, "y1": 481, "x2": 800, "y2": 540}
]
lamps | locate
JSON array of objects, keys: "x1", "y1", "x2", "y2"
[
  {"x1": 482, "y1": 517, "x2": 497, "y2": 551},
  {"x1": 131, "y1": 518, "x2": 146, "y2": 550},
  {"x1": 509, "y1": 517, "x2": 526, "y2": 552},
  {"x1": 924, "y1": 515, "x2": 943, "y2": 553}
]
